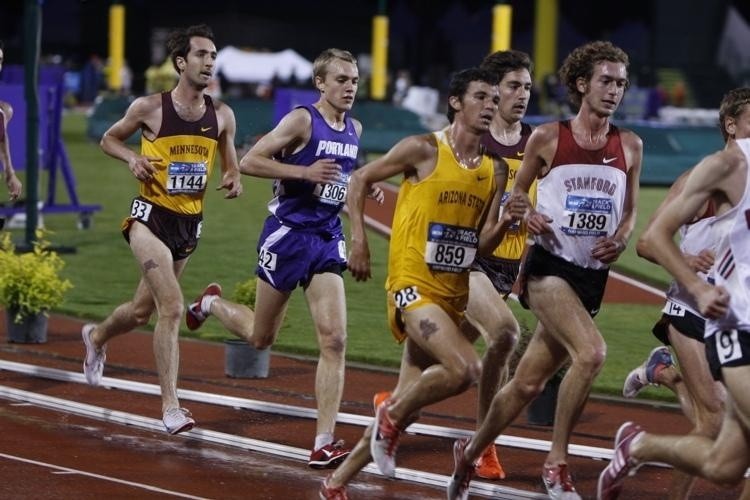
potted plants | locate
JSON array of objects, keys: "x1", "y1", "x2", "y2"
[
  {"x1": 0, "y1": 222, "x2": 76, "y2": 344},
  {"x1": 504, "y1": 313, "x2": 575, "y2": 429},
  {"x1": 221, "y1": 275, "x2": 296, "y2": 380}
]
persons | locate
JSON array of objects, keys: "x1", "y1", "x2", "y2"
[
  {"x1": 597, "y1": 139, "x2": 750, "y2": 500},
  {"x1": 186, "y1": 48, "x2": 386, "y2": 470},
  {"x1": 0, "y1": 47, "x2": 23, "y2": 203},
  {"x1": 83, "y1": 23, "x2": 241, "y2": 435},
  {"x1": 622, "y1": 88, "x2": 750, "y2": 500},
  {"x1": 447, "y1": 41, "x2": 643, "y2": 500},
  {"x1": 318, "y1": 65, "x2": 530, "y2": 500},
  {"x1": 372, "y1": 50, "x2": 542, "y2": 481}
]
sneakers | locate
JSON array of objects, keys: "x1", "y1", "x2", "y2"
[
  {"x1": 597, "y1": 421, "x2": 645, "y2": 498},
  {"x1": 308, "y1": 441, "x2": 349, "y2": 468},
  {"x1": 81, "y1": 323, "x2": 109, "y2": 385},
  {"x1": 540, "y1": 461, "x2": 581, "y2": 500},
  {"x1": 445, "y1": 438, "x2": 475, "y2": 499},
  {"x1": 186, "y1": 283, "x2": 221, "y2": 331},
  {"x1": 318, "y1": 475, "x2": 348, "y2": 500},
  {"x1": 475, "y1": 445, "x2": 505, "y2": 479},
  {"x1": 161, "y1": 409, "x2": 196, "y2": 434},
  {"x1": 370, "y1": 401, "x2": 401, "y2": 479},
  {"x1": 622, "y1": 345, "x2": 675, "y2": 399}
]
